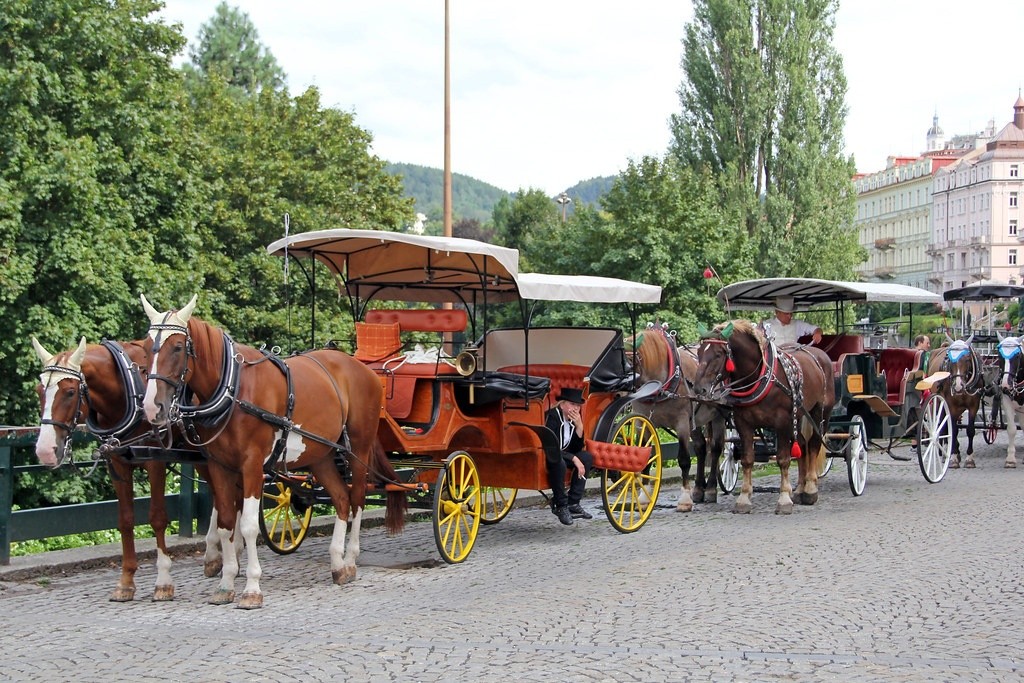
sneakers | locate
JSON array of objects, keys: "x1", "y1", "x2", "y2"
[
  {"x1": 550, "y1": 497, "x2": 573, "y2": 524},
  {"x1": 567, "y1": 503, "x2": 592, "y2": 519},
  {"x1": 909, "y1": 447, "x2": 917, "y2": 453}
]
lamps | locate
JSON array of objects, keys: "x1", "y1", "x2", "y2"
[{"x1": 452, "y1": 341, "x2": 487, "y2": 385}]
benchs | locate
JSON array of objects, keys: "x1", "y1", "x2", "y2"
[
  {"x1": 864, "y1": 345, "x2": 926, "y2": 406},
  {"x1": 461, "y1": 370, "x2": 555, "y2": 456},
  {"x1": 350, "y1": 306, "x2": 468, "y2": 424},
  {"x1": 467, "y1": 327, "x2": 627, "y2": 413},
  {"x1": 797, "y1": 333, "x2": 866, "y2": 383}
]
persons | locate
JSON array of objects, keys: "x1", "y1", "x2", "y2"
[
  {"x1": 1017, "y1": 320, "x2": 1024, "y2": 336},
  {"x1": 544, "y1": 387, "x2": 593, "y2": 525},
  {"x1": 910, "y1": 335, "x2": 930, "y2": 452},
  {"x1": 941, "y1": 342, "x2": 950, "y2": 347},
  {"x1": 762, "y1": 295, "x2": 823, "y2": 346},
  {"x1": 1004, "y1": 321, "x2": 1013, "y2": 331}
]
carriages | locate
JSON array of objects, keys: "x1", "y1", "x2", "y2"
[
  {"x1": 31, "y1": 211, "x2": 665, "y2": 610},
  {"x1": 928, "y1": 280, "x2": 1024, "y2": 468},
  {"x1": 606, "y1": 278, "x2": 953, "y2": 515}
]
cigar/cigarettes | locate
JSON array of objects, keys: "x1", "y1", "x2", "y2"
[{"x1": 582, "y1": 476, "x2": 586, "y2": 480}]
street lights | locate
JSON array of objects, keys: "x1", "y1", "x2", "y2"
[{"x1": 558, "y1": 194, "x2": 572, "y2": 224}]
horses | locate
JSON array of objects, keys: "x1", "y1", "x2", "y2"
[
  {"x1": 624, "y1": 319, "x2": 836, "y2": 516},
  {"x1": 32, "y1": 294, "x2": 409, "y2": 610},
  {"x1": 938, "y1": 330, "x2": 1024, "y2": 468}
]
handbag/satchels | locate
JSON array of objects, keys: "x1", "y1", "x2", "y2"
[{"x1": 383, "y1": 343, "x2": 457, "y2": 373}]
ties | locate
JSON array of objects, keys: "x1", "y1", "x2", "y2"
[{"x1": 563, "y1": 421, "x2": 570, "y2": 447}]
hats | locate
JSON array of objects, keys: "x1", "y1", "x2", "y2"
[
  {"x1": 772, "y1": 296, "x2": 797, "y2": 313},
  {"x1": 556, "y1": 388, "x2": 585, "y2": 404}
]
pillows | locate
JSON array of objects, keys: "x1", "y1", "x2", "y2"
[{"x1": 353, "y1": 320, "x2": 403, "y2": 365}]
